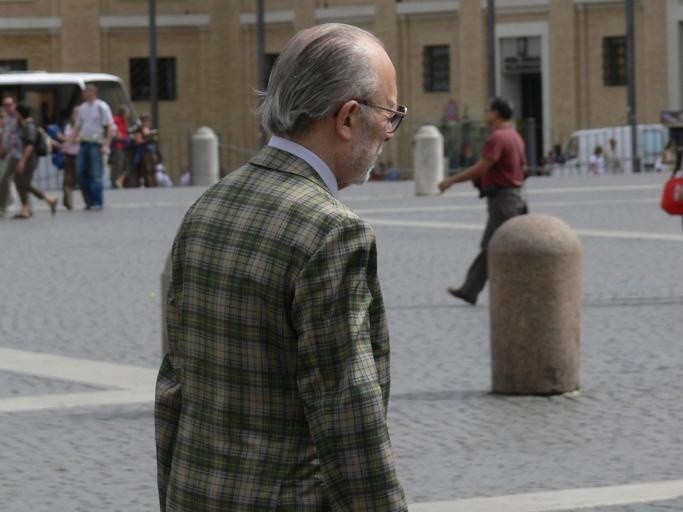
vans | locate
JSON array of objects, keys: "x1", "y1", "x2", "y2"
[{"x1": 544, "y1": 124, "x2": 670, "y2": 174}]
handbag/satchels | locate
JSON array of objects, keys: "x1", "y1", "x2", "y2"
[{"x1": 661, "y1": 161, "x2": 683, "y2": 216}]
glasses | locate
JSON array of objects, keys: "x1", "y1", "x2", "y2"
[{"x1": 350, "y1": 95, "x2": 410, "y2": 134}]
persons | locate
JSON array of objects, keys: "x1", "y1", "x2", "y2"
[
  {"x1": 154, "y1": 20, "x2": 412, "y2": 512},
  {"x1": 540, "y1": 133, "x2": 620, "y2": 174},
  {"x1": 0, "y1": 84, "x2": 162, "y2": 220},
  {"x1": 438, "y1": 96, "x2": 532, "y2": 304},
  {"x1": 658, "y1": 109, "x2": 683, "y2": 216}
]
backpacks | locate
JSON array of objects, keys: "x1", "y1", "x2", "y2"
[{"x1": 34, "y1": 123, "x2": 51, "y2": 156}]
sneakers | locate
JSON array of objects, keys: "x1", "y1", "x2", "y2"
[
  {"x1": 446, "y1": 286, "x2": 479, "y2": 305},
  {"x1": 0, "y1": 178, "x2": 128, "y2": 221}
]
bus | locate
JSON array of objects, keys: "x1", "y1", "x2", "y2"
[{"x1": 0, "y1": 72, "x2": 161, "y2": 186}]
